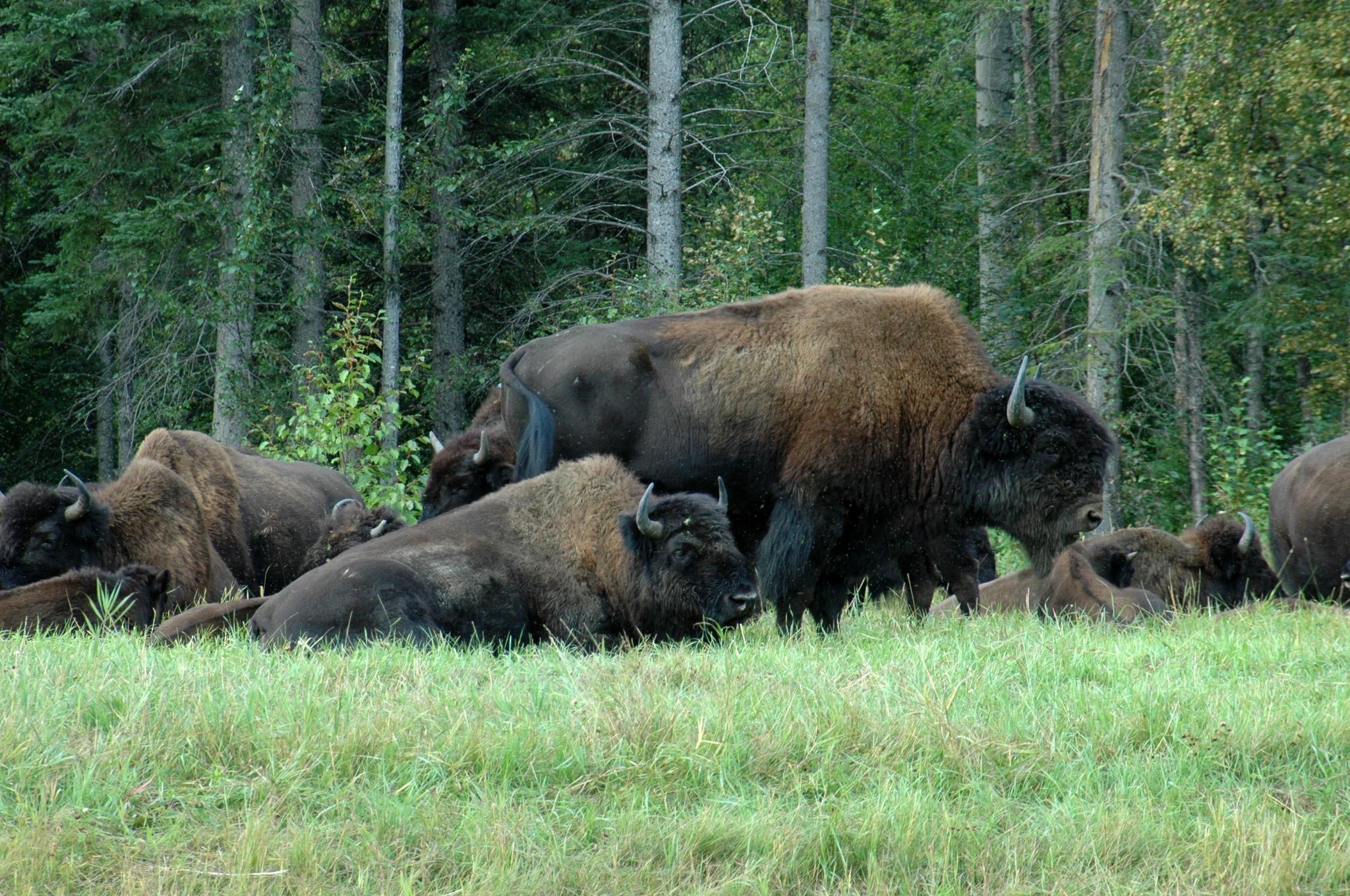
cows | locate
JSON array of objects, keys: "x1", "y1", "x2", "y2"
[
  {"x1": 0, "y1": 427, "x2": 1350, "y2": 653},
  {"x1": 496, "y1": 278, "x2": 1115, "y2": 636}
]
